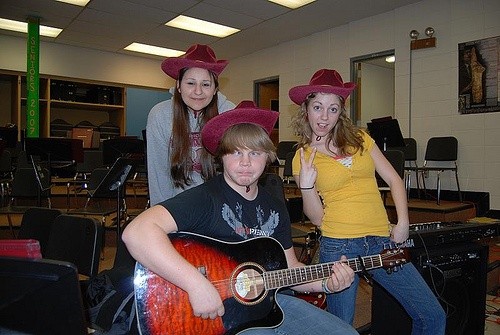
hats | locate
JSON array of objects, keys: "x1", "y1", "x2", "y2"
[
  {"x1": 201, "y1": 100, "x2": 280, "y2": 156},
  {"x1": 289, "y1": 69, "x2": 355, "y2": 106},
  {"x1": 161, "y1": 44, "x2": 228, "y2": 80}
]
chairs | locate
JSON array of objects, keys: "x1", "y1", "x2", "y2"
[{"x1": 0, "y1": 131, "x2": 462, "y2": 335}]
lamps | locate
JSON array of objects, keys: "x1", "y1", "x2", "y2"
[{"x1": 410, "y1": 27, "x2": 436, "y2": 50}]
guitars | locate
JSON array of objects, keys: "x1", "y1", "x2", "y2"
[{"x1": 133, "y1": 231, "x2": 416, "y2": 335}]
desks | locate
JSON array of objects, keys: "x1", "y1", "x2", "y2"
[{"x1": 0, "y1": 205, "x2": 117, "y2": 260}]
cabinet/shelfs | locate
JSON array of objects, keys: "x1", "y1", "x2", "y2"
[{"x1": 0, "y1": 68, "x2": 126, "y2": 150}]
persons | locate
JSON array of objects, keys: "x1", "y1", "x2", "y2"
[
  {"x1": 289, "y1": 69, "x2": 447, "y2": 335},
  {"x1": 146, "y1": 43, "x2": 235, "y2": 210},
  {"x1": 122, "y1": 100, "x2": 360, "y2": 335}
]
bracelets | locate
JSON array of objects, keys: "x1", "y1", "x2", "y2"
[
  {"x1": 300, "y1": 186, "x2": 314, "y2": 189},
  {"x1": 322, "y1": 279, "x2": 333, "y2": 294}
]
